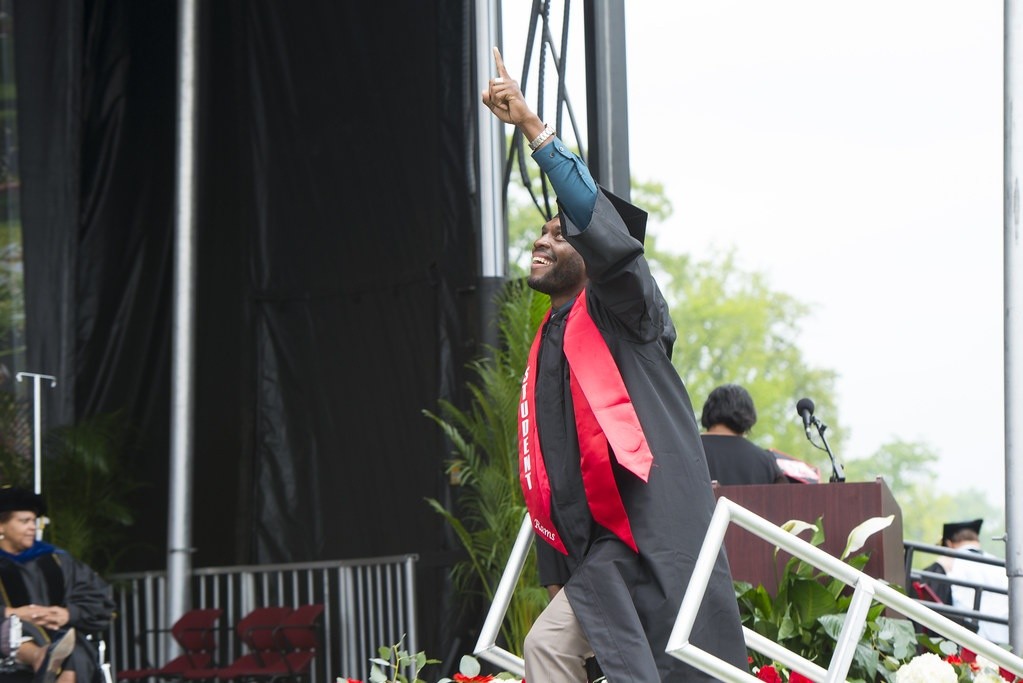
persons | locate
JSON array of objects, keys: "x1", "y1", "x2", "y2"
[
  {"x1": 0, "y1": 485, "x2": 117, "y2": 683},
  {"x1": 698, "y1": 383, "x2": 790, "y2": 484},
  {"x1": 919, "y1": 514, "x2": 1011, "y2": 645},
  {"x1": 482, "y1": 45, "x2": 749, "y2": 682}
]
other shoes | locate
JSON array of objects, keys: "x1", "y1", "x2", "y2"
[{"x1": 35, "y1": 626, "x2": 76, "y2": 683}]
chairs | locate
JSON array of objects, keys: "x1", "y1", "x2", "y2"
[{"x1": 117, "y1": 604, "x2": 325, "y2": 683}]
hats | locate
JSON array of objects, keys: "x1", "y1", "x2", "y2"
[
  {"x1": 599, "y1": 185, "x2": 648, "y2": 246},
  {"x1": 941, "y1": 519, "x2": 983, "y2": 547},
  {"x1": 0, "y1": 487, "x2": 46, "y2": 518}
]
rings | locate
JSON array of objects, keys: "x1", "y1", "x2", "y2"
[{"x1": 494, "y1": 77, "x2": 503, "y2": 85}]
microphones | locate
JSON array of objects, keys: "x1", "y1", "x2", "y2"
[{"x1": 796, "y1": 397, "x2": 815, "y2": 439}]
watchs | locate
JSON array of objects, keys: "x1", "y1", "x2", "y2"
[{"x1": 528, "y1": 121, "x2": 557, "y2": 150}]
